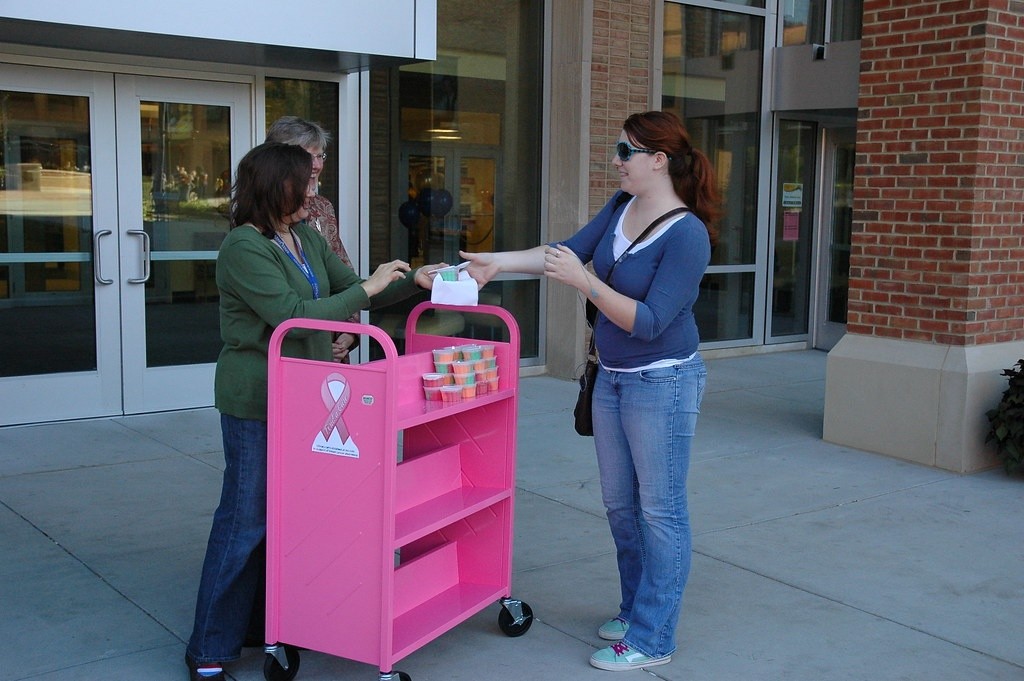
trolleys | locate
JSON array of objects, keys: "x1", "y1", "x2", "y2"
[{"x1": 262, "y1": 300, "x2": 540, "y2": 681}]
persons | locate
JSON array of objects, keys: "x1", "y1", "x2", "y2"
[
  {"x1": 458, "y1": 109, "x2": 729, "y2": 671},
  {"x1": 182, "y1": 139, "x2": 450, "y2": 681},
  {"x1": 264, "y1": 115, "x2": 361, "y2": 363}
]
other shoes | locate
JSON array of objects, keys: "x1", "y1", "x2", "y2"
[
  {"x1": 242, "y1": 635, "x2": 266, "y2": 648},
  {"x1": 185, "y1": 653, "x2": 227, "y2": 681}
]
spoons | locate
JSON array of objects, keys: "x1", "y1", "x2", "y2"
[{"x1": 428, "y1": 261, "x2": 471, "y2": 274}]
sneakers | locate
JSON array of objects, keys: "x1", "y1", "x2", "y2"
[
  {"x1": 589, "y1": 641, "x2": 671, "y2": 672},
  {"x1": 598, "y1": 615, "x2": 630, "y2": 640}
]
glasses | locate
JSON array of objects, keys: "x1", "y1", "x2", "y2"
[
  {"x1": 310, "y1": 152, "x2": 327, "y2": 162},
  {"x1": 616, "y1": 140, "x2": 673, "y2": 163}
]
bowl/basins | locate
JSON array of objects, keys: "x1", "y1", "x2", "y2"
[
  {"x1": 440, "y1": 267, "x2": 458, "y2": 281},
  {"x1": 422, "y1": 343, "x2": 501, "y2": 403}
]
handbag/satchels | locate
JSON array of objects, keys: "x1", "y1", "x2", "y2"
[{"x1": 573, "y1": 358, "x2": 599, "y2": 437}]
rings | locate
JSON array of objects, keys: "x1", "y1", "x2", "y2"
[{"x1": 555, "y1": 250, "x2": 562, "y2": 258}]
